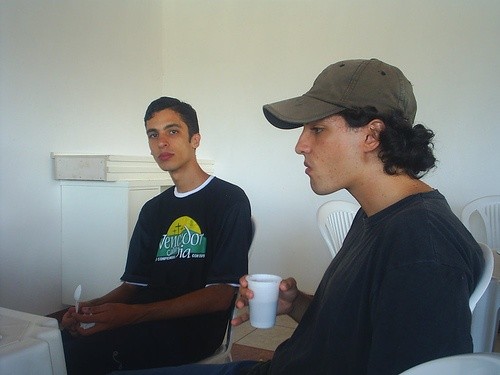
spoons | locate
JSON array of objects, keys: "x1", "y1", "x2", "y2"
[{"x1": 73, "y1": 284, "x2": 81, "y2": 315}]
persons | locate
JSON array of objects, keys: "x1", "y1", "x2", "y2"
[
  {"x1": 59, "y1": 96, "x2": 254, "y2": 375},
  {"x1": 124, "y1": 58, "x2": 487, "y2": 375}
]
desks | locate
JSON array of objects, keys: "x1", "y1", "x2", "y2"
[
  {"x1": 50, "y1": 151, "x2": 216, "y2": 303},
  {"x1": 0, "y1": 307, "x2": 68, "y2": 375}
]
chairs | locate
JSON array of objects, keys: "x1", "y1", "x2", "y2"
[
  {"x1": 465, "y1": 196, "x2": 500, "y2": 352},
  {"x1": 317, "y1": 200, "x2": 360, "y2": 258},
  {"x1": 193, "y1": 217, "x2": 257, "y2": 365}
]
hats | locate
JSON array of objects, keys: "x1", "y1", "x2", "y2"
[{"x1": 262, "y1": 57, "x2": 417, "y2": 130}]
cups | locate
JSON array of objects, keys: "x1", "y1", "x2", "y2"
[{"x1": 245, "y1": 274, "x2": 282, "y2": 329}]
